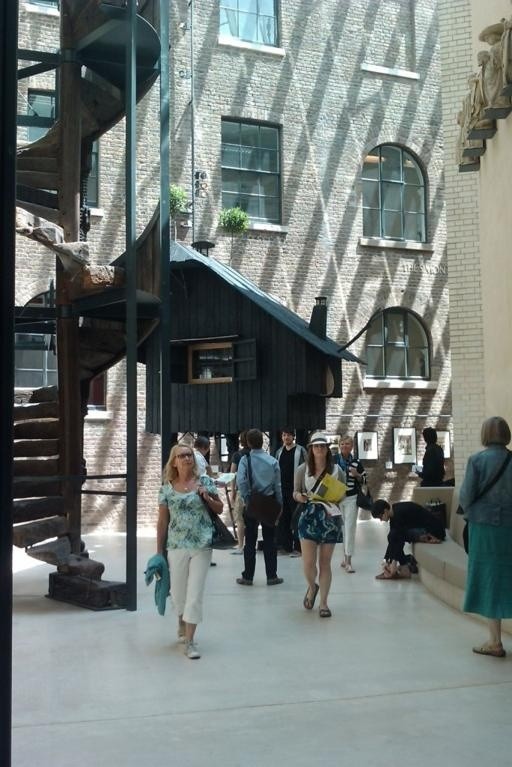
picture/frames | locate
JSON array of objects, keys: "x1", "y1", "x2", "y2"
[
  {"x1": 435, "y1": 429, "x2": 450, "y2": 460},
  {"x1": 356, "y1": 431, "x2": 378, "y2": 460},
  {"x1": 393, "y1": 428, "x2": 418, "y2": 465},
  {"x1": 327, "y1": 434, "x2": 342, "y2": 454}
]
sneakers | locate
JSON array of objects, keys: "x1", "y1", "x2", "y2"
[
  {"x1": 289, "y1": 549, "x2": 300, "y2": 557},
  {"x1": 278, "y1": 547, "x2": 286, "y2": 554},
  {"x1": 185, "y1": 641, "x2": 200, "y2": 658},
  {"x1": 177, "y1": 629, "x2": 186, "y2": 643}
]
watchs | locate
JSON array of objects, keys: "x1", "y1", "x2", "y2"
[{"x1": 207, "y1": 497, "x2": 214, "y2": 505}]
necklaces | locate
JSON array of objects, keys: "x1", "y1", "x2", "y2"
[{"x1": 176, "y1": 477, "x2": 196, "y2": 492}]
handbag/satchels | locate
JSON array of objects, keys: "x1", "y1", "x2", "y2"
[
  {"x1": 462, "y1": 450, "x2": 512, "y2": 555},
  {"x1": 356, "y1": 462, "x2": 373, "y2": 510},
  {"x1": 197, "y1": 478, "x2": 237, "y2": 550},
  {"x1": 291, "y1": 464, "x2": 332, "y2": 532},
  {"x1": 425, "y1": 498, "x2": 446, "y2": 529},
  {"x1": 243, "y1": 454, "x2": 282, "y2": 527}
]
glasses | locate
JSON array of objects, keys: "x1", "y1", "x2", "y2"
[{"x1": 175, "y1": 452, "x2": 192, "y2": 459}]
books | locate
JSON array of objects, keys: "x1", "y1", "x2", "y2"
[
  {"x1": 332, "y1": 435, "x2": 367, "y2": 573},
  {"x1": 307, "y1": 471, "x2": 347, "y2": 517},
  {"x1": 209, "y1": 471, "x2": 235, "y2": 486}
]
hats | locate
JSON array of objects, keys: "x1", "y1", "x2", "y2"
[{"x1": 307, "y1": 432, "x2": 332, "y2": 448}]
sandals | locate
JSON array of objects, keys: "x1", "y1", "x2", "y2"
[
  {"x1": 396, "y1": 571, "x2": 412, "y2": 579},
  {"x1": 473, "y1": 641, "x2": 506, "y2": 657},
  {"x1": 346, "y1": 564, "x2": 353, "y2": 572},
  {"x1": 376, "y1": 568, "x2": 395, "y2": 578},
  {"x1": 341, "y1": 560, "x2": 348, "y2": 568}
]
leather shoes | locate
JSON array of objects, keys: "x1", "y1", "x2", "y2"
[
  {"x1": 266, "y1": 576, "x2": 282, "y2": 585},
  {"x1": 237, "y1": 576, "x2": 253, "y2": 585}
]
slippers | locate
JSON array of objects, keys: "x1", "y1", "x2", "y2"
[
  {"x1": 303, "y1": 584, "x2": 319, "y2": 610},
  {"x1": 319, "y1": 605, "x2": 331, "y2": 619}
]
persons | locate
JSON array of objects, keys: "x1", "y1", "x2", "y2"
[
  {"x1": 293, "y1": 432, "x2": 347, "y2": 618},
  {"x1": 369, "y1": 498, "x2": 447, "y2": 580},
  {"x1": 190, "y1": 436, "x2": 218, "y2": 566},
  {"x1": 412, "y1": 426, "x2": 445, "y2": 488},
  {"x1": 230, "y1": 431, "x2": 250, "y2": 555},
  {"x1": 456, "y1": 418, "x2": 512, "y2": 655},
  {"x1": 235, "y1": 429, "x2": 285, "y2": 586},
  {"x1": 275, "y1": 426, "x2": 308, "y2": 558},
  {"x1": 144, "y1": 445, "x2": 224, "y2": 660}
]
